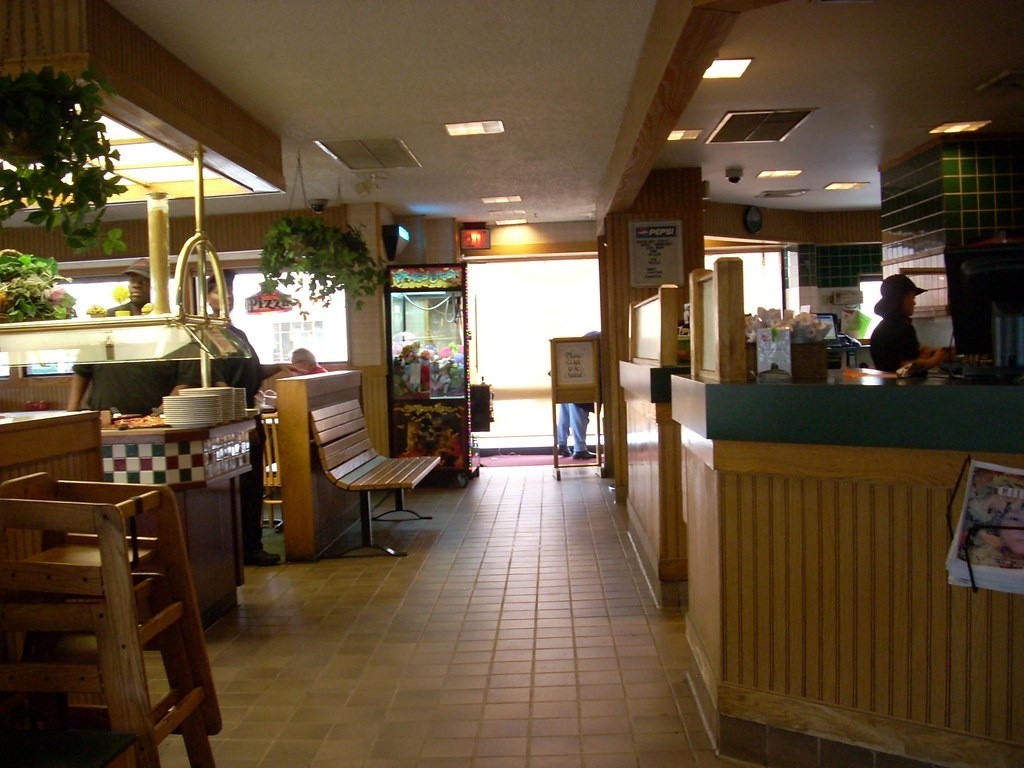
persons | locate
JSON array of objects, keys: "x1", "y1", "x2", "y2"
[
  {"x1": 68, "y1": 258, "x2": 199, "y2": 424},
  {"x1": 869, "y1": 274, "x2": 957, "y2": 377},
  {"x1": 555, "y1": 331, "x2": 602, "y2": 460},
  {"x1": 291, "y1": 348, "x2": 328, "y2": 375},
  {"x1": 201, "y1": 269, "x2": 309, "y2": 566},
  {"x1": 1001, "y1": 500, "x2": 1024, "y2": 554}
]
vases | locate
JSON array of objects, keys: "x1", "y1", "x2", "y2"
[{"x1": 115, "y1": 311, "x2": 130, "y2": 316}]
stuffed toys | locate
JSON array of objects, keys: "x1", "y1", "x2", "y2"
[{"x1": 392, "y1": 341, "x2": 465, "y2": 397}]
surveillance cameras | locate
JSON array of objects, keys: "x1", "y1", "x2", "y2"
[
  {"x1": 310, "y1": 199, "x2": 328, "y2": 214},
  {"x1": 726, "y1": 169, "x2": 744, "y2": 183}
]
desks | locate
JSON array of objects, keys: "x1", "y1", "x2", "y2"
[{"x1": 0, "y1": 410, "x2": 106, "y2": 733}]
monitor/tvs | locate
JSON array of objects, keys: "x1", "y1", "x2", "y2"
[{"x1": 813, "y1": 312, "x2": 839, "y2": 345}]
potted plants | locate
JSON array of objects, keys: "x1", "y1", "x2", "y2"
[{"x1": 258, "y1": 214, "x2": 388, "y2": 321}]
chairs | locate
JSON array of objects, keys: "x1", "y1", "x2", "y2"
[
  {"x1": 260, "y1": 413, "x2": 283, "y2": 533},
  {"x1": 0, "y1": 471, "x2": 222, "y2": 768}
]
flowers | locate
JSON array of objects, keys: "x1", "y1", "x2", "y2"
[
  {"x1": 0, "y1": 253, "x2": 78, "y2": 323},
  {"x1": 111, "y1": 285, "x2": 131, "y2": 311}
]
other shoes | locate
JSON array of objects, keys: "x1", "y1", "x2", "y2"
[
  {"x1": 245, "y1": 552, "x2": 280, "y2": 566},
  {"x1": 573, "y1": 451, "x2": 596, "y2": 459},
  {"x1": 558, "y1": 445, "x2": 572, "y2": 457}
]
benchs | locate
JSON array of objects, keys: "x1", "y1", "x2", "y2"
[{"x1": 308, "y1": 398, "x2": 441, "y2": 560}]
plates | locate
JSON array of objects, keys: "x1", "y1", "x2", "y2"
[{"x1": 163, "y1": 387, "x2": 247, "y2": 428}]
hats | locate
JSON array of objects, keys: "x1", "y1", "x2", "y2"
[
  {"x1": 121, "y1": 259, "x2": 150, "y2": 279},
  {"x1": 875, "y1": 274, "x2": 927, "y2": 316},
  {"x1": 207, "y1": 269, "x2": 236, "y2": 292}
]
